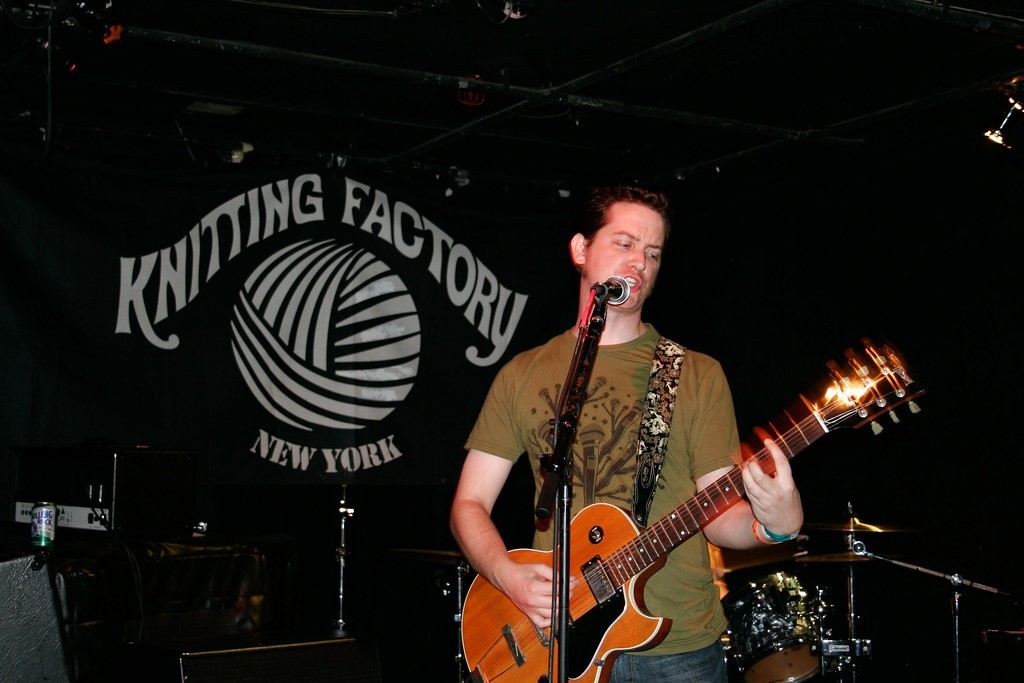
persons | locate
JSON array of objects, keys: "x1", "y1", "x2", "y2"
[{"x1": 449, "y1": 186, "x2": 804, "y2": 683}]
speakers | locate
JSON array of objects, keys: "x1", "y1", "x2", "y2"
[
  {"x1": 178, "y1": 636, "x2": 383, "y2": 683},
  {"x1": 0, "y1": 553, "x2": 71, "y2": 683}
]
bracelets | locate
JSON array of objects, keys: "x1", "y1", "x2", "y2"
[
  {"x1": 762, "y1": 527, "x2": 800, "y2": 543},
  {"x1": 752, "y1": 519, "x2": 778, "y2": 545}
]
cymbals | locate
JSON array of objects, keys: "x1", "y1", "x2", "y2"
[
  {"x1": 802, "y1": 516, "x2": 919, "y2": 533},
  {"x1": 794, "y1": 551, "x2": 908, "y2": 562}
]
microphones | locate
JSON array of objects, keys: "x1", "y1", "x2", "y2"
[{"x1": 590, "y1": 276, "x2": 630, "y2": 306}]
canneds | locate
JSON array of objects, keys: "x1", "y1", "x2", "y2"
[{"x1": 30, "y1": 501, "x2": 57, "y2": 551}]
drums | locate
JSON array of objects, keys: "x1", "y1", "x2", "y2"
[{"x1": 720, "y1": 571, "x2": 821, "y2": 683}]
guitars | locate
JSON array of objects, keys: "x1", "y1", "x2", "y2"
[{"x1": 461, "y1": 344, "x2": 926, "y2": 683}]
